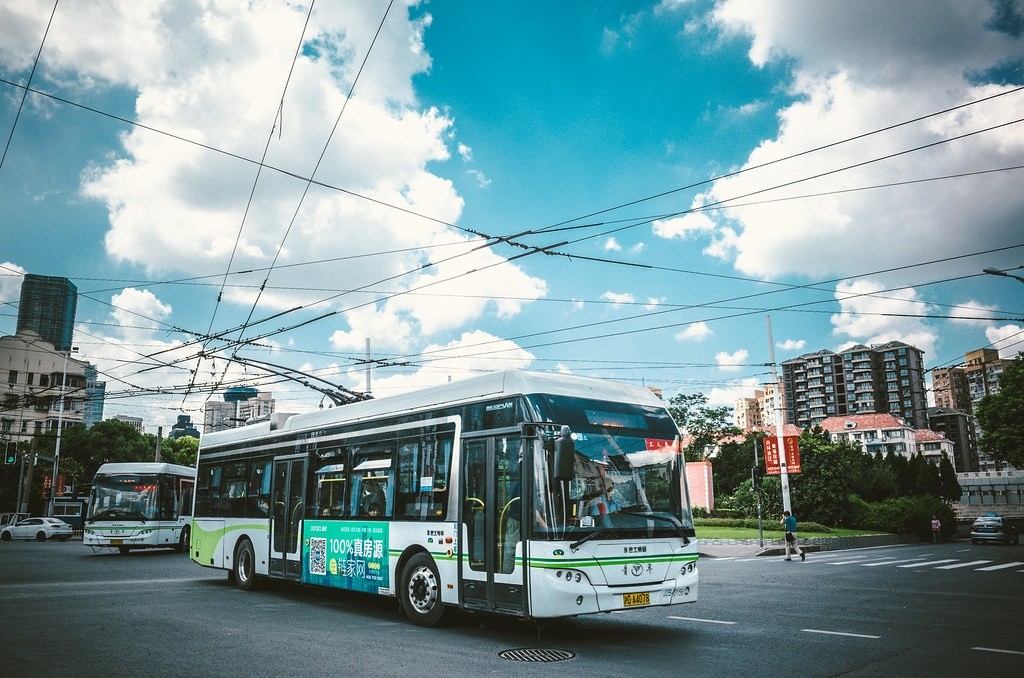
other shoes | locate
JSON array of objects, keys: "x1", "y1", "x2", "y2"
[
  {"x1": 802, "y1": 553, "x2": 805, "y2": 561},
  {"x1": 784, "y1": 558, "x2": 791, "y2": 561}
]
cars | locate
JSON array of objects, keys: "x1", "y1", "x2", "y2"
[
  {"x1": 971, "y1": 512, "x2": 1019, "y2": 545},
  {"x1": 0, "y1": 517, "x2": 73, "y2": 542}
]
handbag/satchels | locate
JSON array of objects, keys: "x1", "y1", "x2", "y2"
[
  {"x1": 786, "y1": 533, "x2": 794, "y2": 542},
  {"x1": 932, "y1": 528, "x2": 937, "y2": 532}
]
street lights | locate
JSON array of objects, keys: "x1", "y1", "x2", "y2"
[{"x1": 752, "y1": 426, "x2": 764, "y2": 546}]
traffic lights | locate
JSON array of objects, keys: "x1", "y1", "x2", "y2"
[
  {"x1": 5, "y1": 441, "x2": 17, "y2": 465},
  {"x1": 752, "y1": 465, "x2": 764, "y2": 491}
]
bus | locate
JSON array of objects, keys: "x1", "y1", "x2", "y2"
[
  {"x1": 83, "y1": 463, "x2": 196, "y2": 555},
  {"x1": 189, "y1": 368, "x2": 699, "y2": 628}
]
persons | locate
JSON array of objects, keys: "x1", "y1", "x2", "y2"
[
  {"x1": 322, "y1": 506, "x2": 332, "y2": 517},
  {"x1": 362, "y1": 470, "x2": 386, "y2": 516},
  {"x1": 588, "y1": 477, "x2": 621, "y2": 515},
  {"x1": 931, "y1": 514, "x2": 941, "y2": 544},
  {"x1": 780, "y1": 510, "x2": 805, "y2": 561}
]
